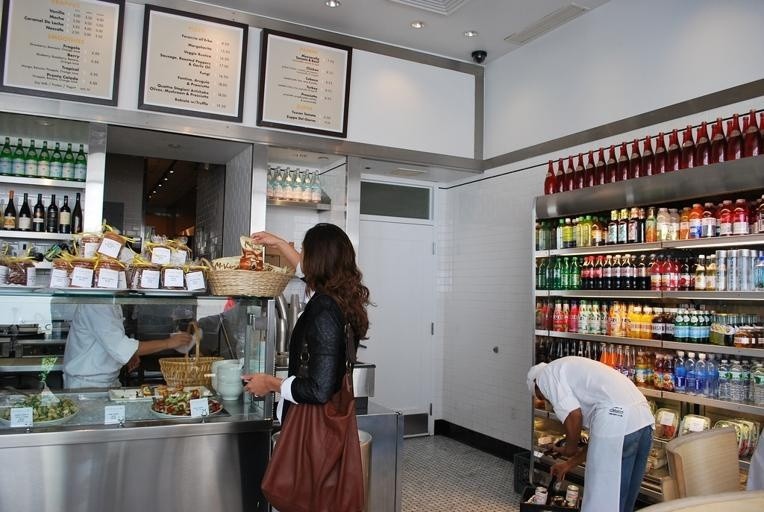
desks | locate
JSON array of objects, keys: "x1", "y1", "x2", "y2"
[{"x1": 634, "y1": 490, "x2": 764, "y2": 512}]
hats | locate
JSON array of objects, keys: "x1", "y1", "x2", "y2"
[{"x1": 527, "y1": 362, "x2": 547, "y2": 400}]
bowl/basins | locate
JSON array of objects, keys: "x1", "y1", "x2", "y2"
[{"x1": 210, "y1": 358, "x2": 243, "y2": 401}]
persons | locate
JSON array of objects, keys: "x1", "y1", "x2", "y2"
[
  {"x1": 62, "y1": 305, "x2": 192, "y2": 388},
  {"x1": 525, "y1": 355, "x2": 656, "y2": 512},
  {"x1": 240, "y1": 224, "x2": 378, "y2": 511}
]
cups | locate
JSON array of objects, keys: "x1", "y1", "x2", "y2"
[{"x1": 270, "y1": 428, "x2": 372, "y2": 512}]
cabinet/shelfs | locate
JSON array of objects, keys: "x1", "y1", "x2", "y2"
[
  {"x1": 529, "y1": 154, "x2": 764, "y2": 486},
  {"x1": 0, "y1": 286, "x2": 277, "y2": 512},
  {"x1": 0, "y1": 175, "x2": 85, "y2": 270}
]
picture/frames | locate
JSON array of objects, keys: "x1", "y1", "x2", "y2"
[
  {"x1": 256, "y1": 27, "x2": 352, "y2": 138},
  {"x1": 0, "y1": 0, "x2": 126, "y2": 107},
  {"x1": 137, "y1": 3, "x2": 249, "y2": 123}
]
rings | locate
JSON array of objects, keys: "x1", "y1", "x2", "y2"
[{"x1": 254, "y1": 395, "x2": 259, "y2": 398}]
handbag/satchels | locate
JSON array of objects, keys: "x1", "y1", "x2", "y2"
[{"x1": 260, "y1": 322, "x2": 365, "y2": 512}]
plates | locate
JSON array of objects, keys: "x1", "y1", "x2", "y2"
[
  {"x1": 108, "y1": 385, "x2": 214, "y2": 403},
  {"x1": 145, "y1": 398, "x2": 225, "y2": 418},
  {"x1": 2, "y1": 397, "x2": 80, "y2": 430}
]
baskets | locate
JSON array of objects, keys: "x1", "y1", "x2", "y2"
[
  {"x1": 158, "y1": 322, "x2": 225, "y2": 388},
  {"x1": 200, "y1": 257, "x2": 295, "y2": 298}
]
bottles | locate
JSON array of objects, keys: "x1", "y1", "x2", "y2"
[
  {"x1": 543, "y1": 108, "x2": 764, "y2": 195},
  {"x1": 535, "y1": 335, "x2": 763, "y2": 407},
  {"x1": 266, "y1": 163, "x2": 322, "y2": 204},
  {"x1": 536, "y1": 248, "x2": 763, "y2": 292},
  {"x1": 0, "y1": 132, "x2": 86, "y2": 183},
  {"x1": 534, "y1": 196, "x2": 764, "y2": 251},
  {"x1": 0, "y1": 186, "x2": 85, "y2": 235},
  {"x1": 533, "y1": 482, "x2": 579, "y2": 507},
  {"x1": 535, "y1": 297, "x2": 764, "y2": 348}
]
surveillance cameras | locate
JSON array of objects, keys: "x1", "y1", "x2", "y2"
[{"x1": 472, "y1": 50, "x2": 487, "y2": 64}]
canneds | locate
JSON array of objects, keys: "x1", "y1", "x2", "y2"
[
  {"x1": 534, "y1": 486, "x2": 548, "y2": 505},
  {"x1": 566, "y1": 484, "x2": 579, "y2": 507}
]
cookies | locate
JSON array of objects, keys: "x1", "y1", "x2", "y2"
[{"x1": 51, "y1": 232, "x2": 208, "y2": 290}]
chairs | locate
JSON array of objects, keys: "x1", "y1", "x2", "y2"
[{"x1": 668, "y1": 427, "x2": 741, "y2": 499}]
